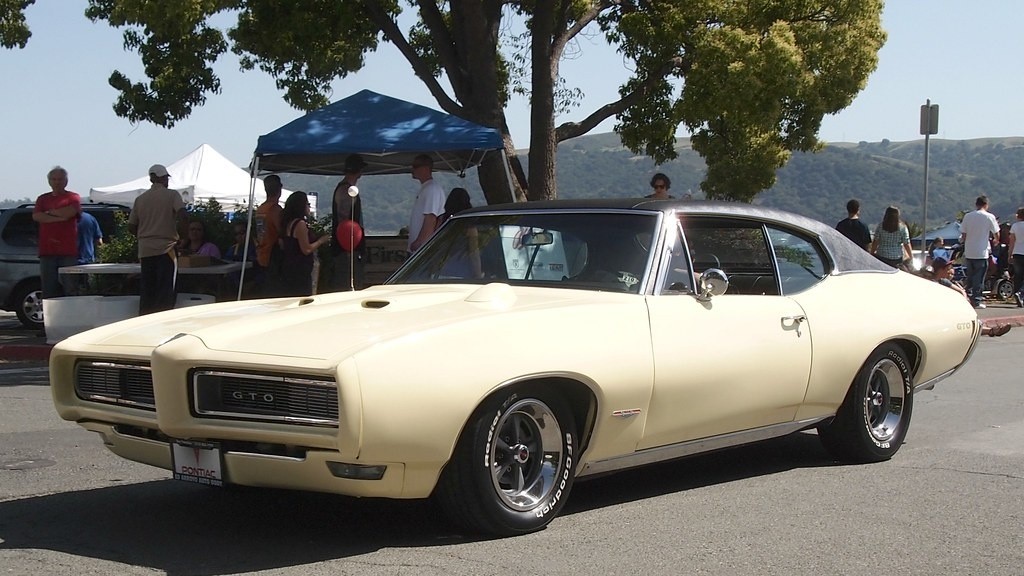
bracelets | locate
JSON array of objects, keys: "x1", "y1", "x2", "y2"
[{"x1": 48, "y1": 208, "x2": 52, "y2": 214}]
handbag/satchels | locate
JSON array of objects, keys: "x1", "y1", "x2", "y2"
[
  {"x1": 269, "y1": 233, "x2": 287, "y2": 278},
  {"x1": 268, "y1": 217, "x2": 314, "y2": 284},
  {"x1": 440, "y1": 231, "x2": 470, "y2": 277}
]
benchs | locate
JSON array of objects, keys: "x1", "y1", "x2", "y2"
[{"x1": 724, "y1": 273, "x2": 811, "y2": 295}]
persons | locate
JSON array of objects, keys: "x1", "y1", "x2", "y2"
[
  {"x1": 872, "y1": 206, "x2": 912, "y2": 270},
  {"x1": 129, "y1": 164, "x2": 188, "y2": 317},
  {"x1": 254, "y1": 176, "x2": 290, "y2": 294},
  {"x1": 643, "y1": 173, "x2": 676, "y2": 199},
  {"x1": 32, "y1": 169, "x2": 81, "y2": 336},
  {"x1": 835, "y1": 200, "x2": 871, "y2": 252},
  {"x1": 179, "y1": 221, "x2": 222, "y2": 294},
  {"x1": 76, "y1": 212, "x2": 102, "y2": 263},
  {"x1": 407, "y1": 155, "x2": 445, "y2": 282},
  {"x1": 224, "y1": 221, "x2": 257, "y2": 286},
  {"x1": 333, "y1": 155, "x2": 365, "y2": 290},
  {"x1": 961, "y1": 196, "x2": 1000, "y2": 308},
  {"x1": 1009, "y1": 208, "x2": 1024, "y2": 307},
  {"x1": 920, "y1": 222, "x2": 1011, "y2": 304},
  {"x1": 434, "y1": 187, "x2": 482, "y2": 280},
  {"x1": 278, "y1": 190, "x2": 332, "y2": 296}
]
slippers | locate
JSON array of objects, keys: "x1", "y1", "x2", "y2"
[{"x1": 996, "y1": 323, "x2": 1011, "y2": 336}]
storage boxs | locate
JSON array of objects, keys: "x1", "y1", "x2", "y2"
[
  {"x1": 365, "y1": 239, "x2": 409, "y2": 272},
  {"x1": 179, "y1": 254, "x2": 211, "y2": 267}
]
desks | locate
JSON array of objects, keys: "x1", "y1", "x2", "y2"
[{"x1": 56, "y1": 258, "x2": 249, "y2": 276}]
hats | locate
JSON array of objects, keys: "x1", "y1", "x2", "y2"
[
  {"x1": 150, "y1": 165, "x2": 170, "y2": 177},
  {"x1": 932, "y1": 257, "x2": 955, "y2": 272},
  {"x1": 344, "y1": 155, "x2": 367, "y2": 175}
]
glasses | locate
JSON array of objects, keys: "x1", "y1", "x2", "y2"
[
  {"x1": 278, "y1": 184, "x2": 283, "y2": 187},
  {"x1": 654, "y1": 185, "x2": 664, "y2": 189},
  {"x1": 306, "y1": 202, "x2": 310, "y2": 207},
  {"x1": 236, "y1": 231, "x2": 246, "y2": 236},
  {"x1": 413, "y1": 164, "x2": 426, "y2": 168}
]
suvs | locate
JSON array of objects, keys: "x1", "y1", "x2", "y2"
[{"x1": 0, "y1": 203, "x2": 133, "y2": 330}]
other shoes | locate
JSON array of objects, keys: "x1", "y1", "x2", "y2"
[
  {"x1": 975, "y1": 304, "x2": 986, "y2": 308},
  {"x1": 1014, "y1": 292, "x2": 1023, "y2": 307}
]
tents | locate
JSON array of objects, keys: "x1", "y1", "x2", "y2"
[
  {"x1": 236, "y1": 90, "x2": 534, "y2": 301},
  {"x1": 89, "y1": 145, "x2": 317, "y2": 220},
  {"x1": 910, "y1": 222, "x2": 961, "y2": 245}
]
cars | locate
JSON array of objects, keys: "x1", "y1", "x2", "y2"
[
  {"x1": 911, "y1": 250, "x2": 1015, "y2": 300},
  {"x1": 49, "y1": 197, "x2": 979, "y2": 536}
]
